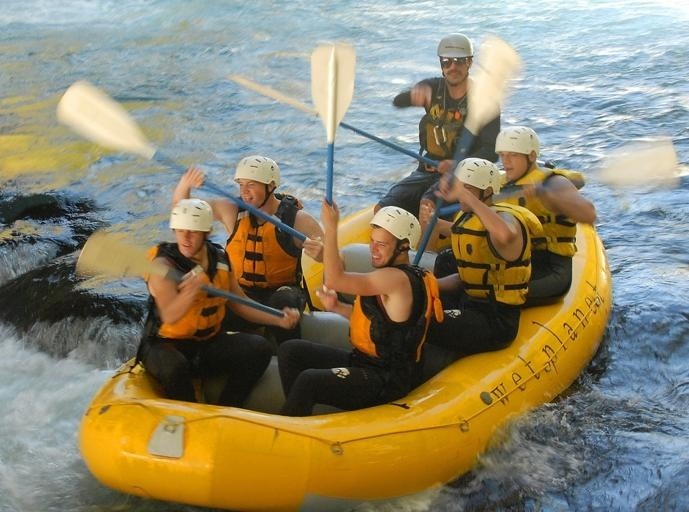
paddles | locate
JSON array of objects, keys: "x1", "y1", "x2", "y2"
[
  {"x1": 411, "y1": 32, "x2": 517, "y2": 267},
  {"x1": 57, "y1": 80, "x2": 310, "y2": 241},
  {"x1": 235, "y1": 73, "x2": 437, "y2": 164},
  {"x1": 434, "y1": 140, "x2": 680, "y2": 217},
  {"x1": 309, "y1": 43, "x2": 355, "y2": 204},
  {"x1": 77, "y1": 232, "x2": 285, "y2": 318}
]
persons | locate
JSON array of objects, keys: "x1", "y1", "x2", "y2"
[
  {"x1": 374, "y1": 33, "x2": 500, "y2": 254},
  {"x1": 437, "y1": 125, "x2": 597, "y2": 307},
  {"x1": 172, "y1": 152, "x2": 326, "y2": 351},
  {"x1": 138, "y1": 200, "x2": 301, "y2": 404},
  {"x1": 275, "y1": 195, "x2": 438, "y2": 415},
  {"x1": 424, "y1": 155, "x2": 529, "y2": 353}
]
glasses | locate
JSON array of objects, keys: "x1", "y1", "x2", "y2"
[{"x1": 439, "y1": 57, "x2": 470, "y2": 67}]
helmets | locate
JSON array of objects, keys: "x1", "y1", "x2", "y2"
[
  {"x1": 167, "y1": 198, "x2": 215, "y2": 233},
  {"x1": 451, "y1": 157, "x2": 501, "y2": 196},
  {"x1": 233, "y1": 154, "x2": 281, "y2": 188},
  {"x1": 369, "y1": 205, "x2": 422, "y2": 252},
  {"x1": 436, "y1": 33, "x2": 476, "y2": 58},
  {"x1": 494, "y1": 125, "x2": 541, "y2": 156}
]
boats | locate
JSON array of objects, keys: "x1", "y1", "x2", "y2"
[{"x1": 76, "y1": 202, "x2": 615, "y2": 507}]
